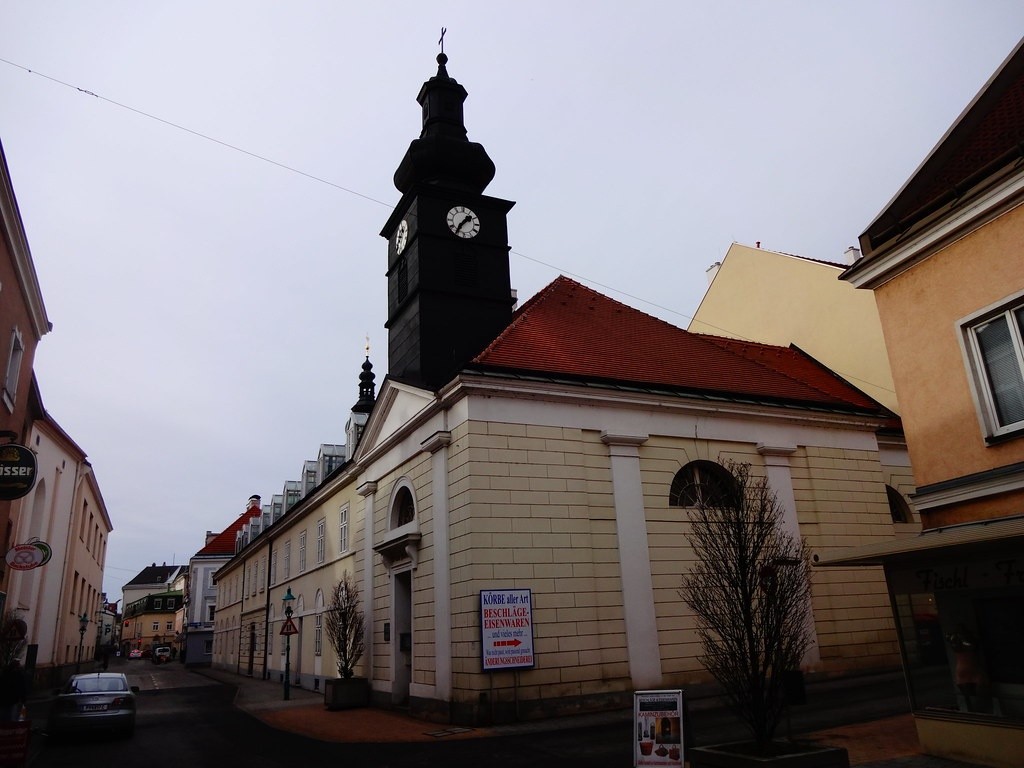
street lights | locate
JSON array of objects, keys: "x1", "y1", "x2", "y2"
[
  {"x1": 75, "y1": 612, "x2": 89, "y2": 672},
  {"x1": 282, "y1": 586, "x2": 296, "y2": 702}
]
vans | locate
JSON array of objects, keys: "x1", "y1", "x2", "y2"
[{"x1": 152, "y1": 647, "x2": 170, "y2": 665}]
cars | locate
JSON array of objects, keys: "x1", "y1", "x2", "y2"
[
  {"x1": 45, "y1": 672, "x2": 139, "y2": 738},
  {"x1": 128, "y1": 649, "x2": 141, "y2": 660}
]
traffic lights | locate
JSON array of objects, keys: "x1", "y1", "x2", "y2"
[
  {"x1": 125, "y1": 621, "x2": 129, "y2": 627},
  {"x1": 138, "y1": 640, "x2": 141, "y2": 645}
]
